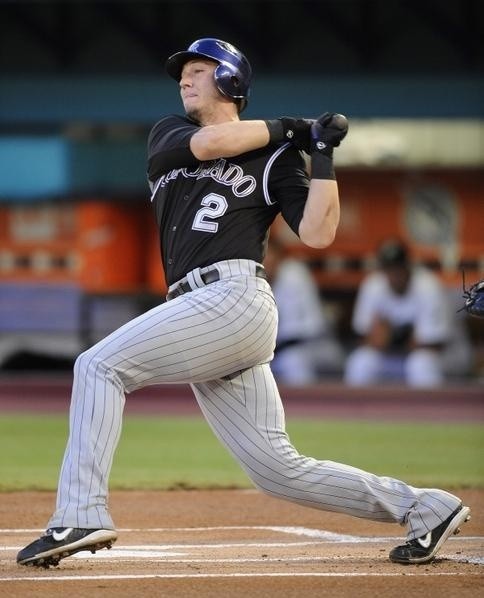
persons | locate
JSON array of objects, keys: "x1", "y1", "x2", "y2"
[
  {"x1": 9, "y1": 34, "x2": 474, "y2": 570},
  {"x1": 340, "y1": 238, "x2": 472, "y2": 392},
  {"x1": 256, "y1": 231, "x2": 342, "y2": 389}
]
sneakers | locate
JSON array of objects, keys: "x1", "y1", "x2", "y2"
[
  {"x1": 388, "y1": 491, "x2": 472, "y2": 565},
  {"x1": 17, "y1": 523, "x2": 118, "y2": 568}
]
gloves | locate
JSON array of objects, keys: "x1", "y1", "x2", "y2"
[{"x1": 279, "y1": 110, "x2": 348, "y2": 159}]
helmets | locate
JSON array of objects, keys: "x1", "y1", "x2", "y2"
[
  {"x1": 164, "y1": 37, "x2": 253, "y2": 112},
  {"x1": 375, "y1": 238, "x2": 412, "y2": 267}
]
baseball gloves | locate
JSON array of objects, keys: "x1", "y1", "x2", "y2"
[{"x1": 457, "y1": 269, "x2": 483, "y2": 318}]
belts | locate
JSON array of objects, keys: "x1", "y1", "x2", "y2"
[{"x1": 166, "y1": 265, "x2": 267, "y2": 300}]
